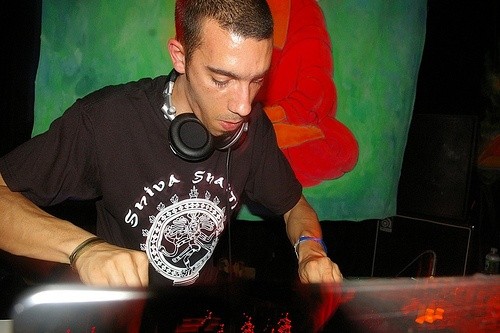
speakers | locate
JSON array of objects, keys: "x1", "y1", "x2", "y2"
[{"x1": 396, "y1": 113, "x2": 480, "y2": 223}]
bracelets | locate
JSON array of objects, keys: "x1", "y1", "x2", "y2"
[
  {"x1": 294, "y1": 236, "x2": 328, "y2": 259},
  {"x1": 69, "y1": 236, "x2": 106, "y2": 273}
]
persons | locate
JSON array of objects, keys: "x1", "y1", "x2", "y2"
[{"x1": 0, "y1": 0, "x2": 345, "y2": 289}]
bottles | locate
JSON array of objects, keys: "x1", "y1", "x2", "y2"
[{"x1": 485, "y1": 248, "x2": 500, "y2": 275}]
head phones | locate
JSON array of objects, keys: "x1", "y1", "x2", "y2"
[{"x1": 161, "y1": 68, "x2": 248, "y2": 163}]
